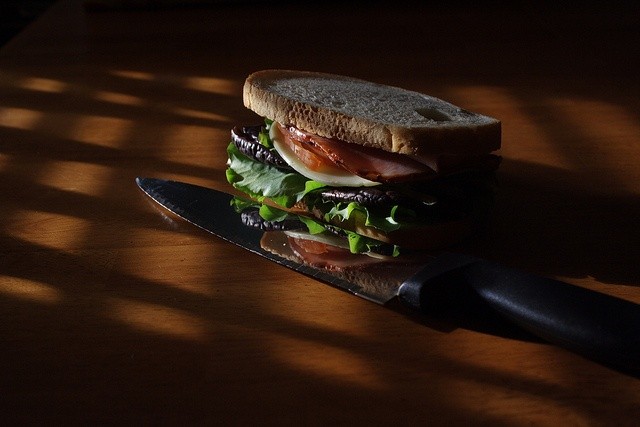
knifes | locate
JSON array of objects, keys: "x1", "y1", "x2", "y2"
[{"x1": 134, "y1": 175, "x2": 639, "y2": 377}]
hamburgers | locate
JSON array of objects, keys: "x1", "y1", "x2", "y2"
[{"x1": 223, "y1": 69, "x2": 503, "y2": 244}]
